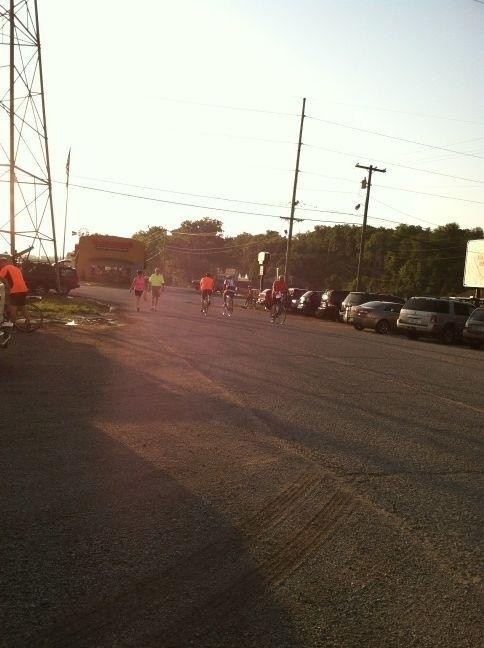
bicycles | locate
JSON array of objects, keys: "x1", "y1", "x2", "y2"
[
  {"x1": 0, "y1": 293, "x2": 45, "y2": 333},
  {"x1": 240, "y1": 287, "x2": 257, "y2": 312},
  {"x1": 223, "y1": 290, "x2": 236, "y2": 317},
  {"x1": 200, "y1": 291, "x2": 212, "y2": 318}
]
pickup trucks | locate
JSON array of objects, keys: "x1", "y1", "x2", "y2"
[{"x1": 191, "y1": 273, "x2": 228, "y2": 295}]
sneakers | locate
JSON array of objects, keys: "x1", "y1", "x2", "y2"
[
  {"x1": 14, "y1": 316, "x2": 27, "y2": 322},
  {"x1": 0, "y1": 319, "x2": 13, "y2": 327}
]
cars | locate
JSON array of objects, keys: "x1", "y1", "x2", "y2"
[{"x1": 249, "y1": 287, "x2": 483, "y2": 349}]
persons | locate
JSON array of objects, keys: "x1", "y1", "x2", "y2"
[
  {"x1": 147, "y1": 267, "x2": 166, "y2": 310},
  {"x1": 221, "y1": 274, "x2": 237, "y2": 312},
  {"x1": 0, "y1": 257, "x2": 31, "y2": 327},
  {"x1": 270, "y1": 275, "x2": 286, "y2": 322},
  {"x1": 198, "y1": 271, "x2": 215, "y2": 312},
  {"x1": 127, "y1": 270, "x2": 145, "y2": 311}
]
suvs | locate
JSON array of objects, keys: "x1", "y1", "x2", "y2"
[{"x1": 21, "y1": 259, "x2": 81, "y2": 295}]
